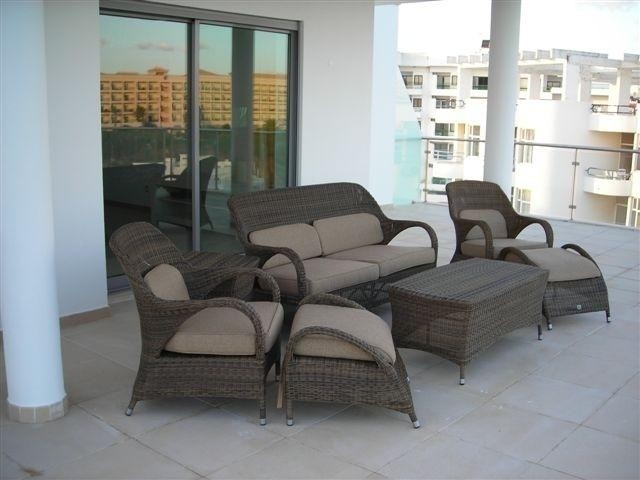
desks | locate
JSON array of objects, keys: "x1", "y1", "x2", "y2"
[{"x1": 181, "y1": 249, "x2": 261, "y2": 302}]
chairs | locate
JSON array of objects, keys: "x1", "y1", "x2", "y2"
[
  {"x1": 157, "y1": 155, "x2": 216, "y2": 235},
  {"x1": 445, "y1": 178, "x2": 554, "y2": 258},
  {"x1": 107, "y1": 219, "x2": 279, "y2": 427}
]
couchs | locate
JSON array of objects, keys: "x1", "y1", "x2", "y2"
[
  {"x1": 100, "y1": 162, "x2": 164, "y2": 227},
  {"x1": 228, "y1": 182, "x2": 439, "y2": 311}
]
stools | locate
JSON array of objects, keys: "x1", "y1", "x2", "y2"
[
  {"x1": 280, "y1": 293, "x2": 422, "y2": 430},
  {"x1": 497, "y1": 243, "x2": 611, "y2": 330}
]
